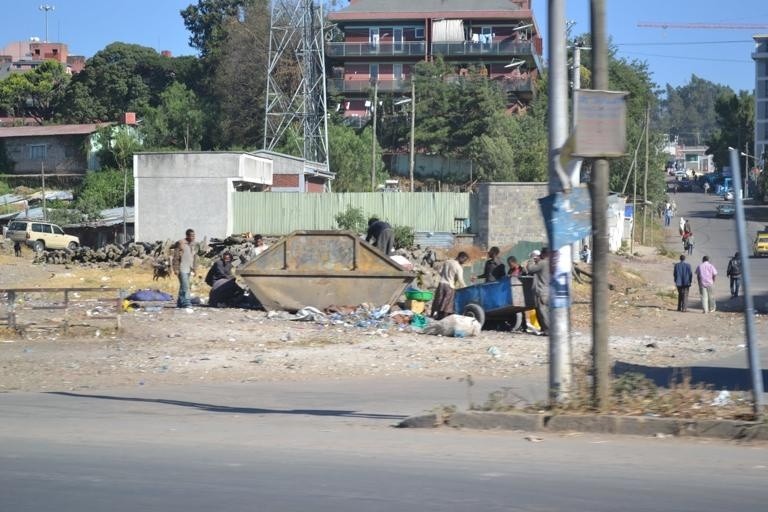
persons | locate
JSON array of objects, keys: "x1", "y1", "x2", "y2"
[
  {"x1": 677, "y1": 216, "x2": 685, "y2": 237},
  {"x1": 673, "y1": 254, "x2": 692, "y2": 314},
  {"x1": 469, "y1": 245, "x2": 504, "y2": 284},
  {"x1": 663, "y1": 199, "x2": 672, "y2": 226},
  {"x1": 579, "y1": 243, "x2": 590, "y2": 263},
  {"x1": 247, "y1": 233, "x2": 268, "y2": 262},
  {"x1": 686, "y1": 232, "x2": 694, "y2": 256},
  {"x1": 204, "y1": 251, "x2": 235, "y2": 288},
  {"x1": 430, "y1": 250, "x2": 469, "y2": 320},
  {"x1": 725, "y1": 250, "x2": 741, "y2": 301},
  {"x1": 363, "y1": 217, "x2": 396, "y2": 256},
  {"x1": 168, "y1": 227, "x2": 197, "y2": 308},
  {"x1": 530, "y1": 250, "x2": 542, "y2": 263},
  {"x1": 681, "y1": 230, "x2": 689, "y2": 253},
  {"x1": 671, "y1": 200, "x2": 677, "y2": 216},
  {"x1": 506, "y1": 255, "x2": 523, "y2": 275},
  {"x1": 524, "y1": 246, "x2": 551, "y2": 337},
  {"x1": 683, "y1": 219, "x2": 691, "y2": 234},
  {"x1": 703, "y1": 180, "x2": 711, "y2": 196},
  {"x1": 694, "y1": 255, "x2": 718, "y2": 314}
]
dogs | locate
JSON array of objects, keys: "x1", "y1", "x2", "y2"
[
  {"x1": 14, "y1": 243, "x2": 22, "y2": 257},
  {"x1": 153, "y1": 266, "x2": 171, "y2": 282}
]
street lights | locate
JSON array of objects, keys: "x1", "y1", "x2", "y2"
[
  {"x1": 727, "y1": 146, "x2": 762, "y2": 160},
  {"x1": 39, "y1": 3, "x2": 56, "y2": 43}
]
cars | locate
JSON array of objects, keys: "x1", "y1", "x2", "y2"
[
  {"x1": 716, "y1": 203, "x2": 736, "y2": 216},
  {"x1": 667, "y1": 167, "x2": 693, "y2": 193},
  {"x1": 752, "y1": 225, "x2": 767, "y2": 258}
]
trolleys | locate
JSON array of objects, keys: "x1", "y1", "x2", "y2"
[{"x1": 449, "y1": 272, "x2": 538, "y2": 334}]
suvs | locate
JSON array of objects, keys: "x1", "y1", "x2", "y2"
[{"x1": 6, "y1": 217, "x2": 82, "y2": 253}]
